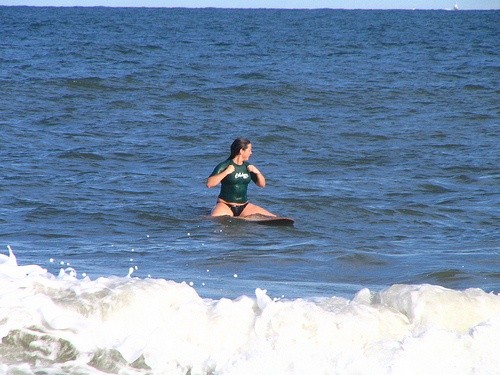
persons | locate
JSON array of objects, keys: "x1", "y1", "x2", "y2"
[{"x1": 205, "y1": 137, "x2": 277, "y2": 218}]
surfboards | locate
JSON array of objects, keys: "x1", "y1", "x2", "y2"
[{"x1": 234, "y1": 217, "x2": 295, "y2": 226}]
